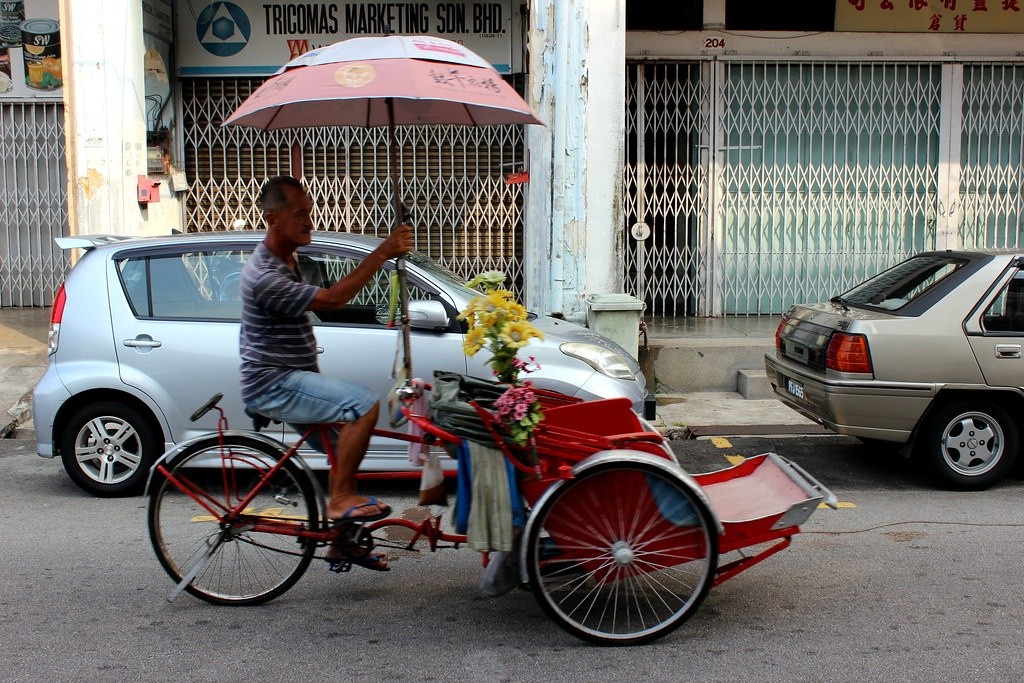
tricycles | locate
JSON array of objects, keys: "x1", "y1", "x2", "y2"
[{"x1": 146, "y1": 34, "x2": 837, "y2": 646}]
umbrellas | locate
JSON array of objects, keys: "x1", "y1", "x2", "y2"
[{"x1": 218, "y1": 36, "x2": 547, "y2": 383}]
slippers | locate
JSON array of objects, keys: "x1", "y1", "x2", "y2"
[
  {"x1": 325, "y1": 552, "x2": 390, "y2": 571},
  {"x1": 332, "y1": 497, "x2": 392, "y2": 527}
]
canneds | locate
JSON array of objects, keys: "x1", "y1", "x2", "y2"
[{"x1": 0, "y1": 0, "x2": 64, "y2": 92}]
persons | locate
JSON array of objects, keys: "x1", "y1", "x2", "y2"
[{"x1": 238, "y1": 175, "x2": 414, "y2": 572}]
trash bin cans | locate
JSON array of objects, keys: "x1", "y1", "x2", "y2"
[{"x1": 584, "y1": 292, "x2": 648, "y2": 365}]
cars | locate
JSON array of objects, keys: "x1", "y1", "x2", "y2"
[
  {"x1": 32, "y1": 229, "x2": 649, "y2": 496},
  {"x1": 764, "y1": 250, "x2": 1024, "y2": 490}
]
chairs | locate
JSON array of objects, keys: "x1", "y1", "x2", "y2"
[{"x1": 540, "y1": 397, "x2": 670, "y2": 459}]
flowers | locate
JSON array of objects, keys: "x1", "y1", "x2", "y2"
[{"x1": 455, "y1": 270, "x2": 545, "y2": 448}]
